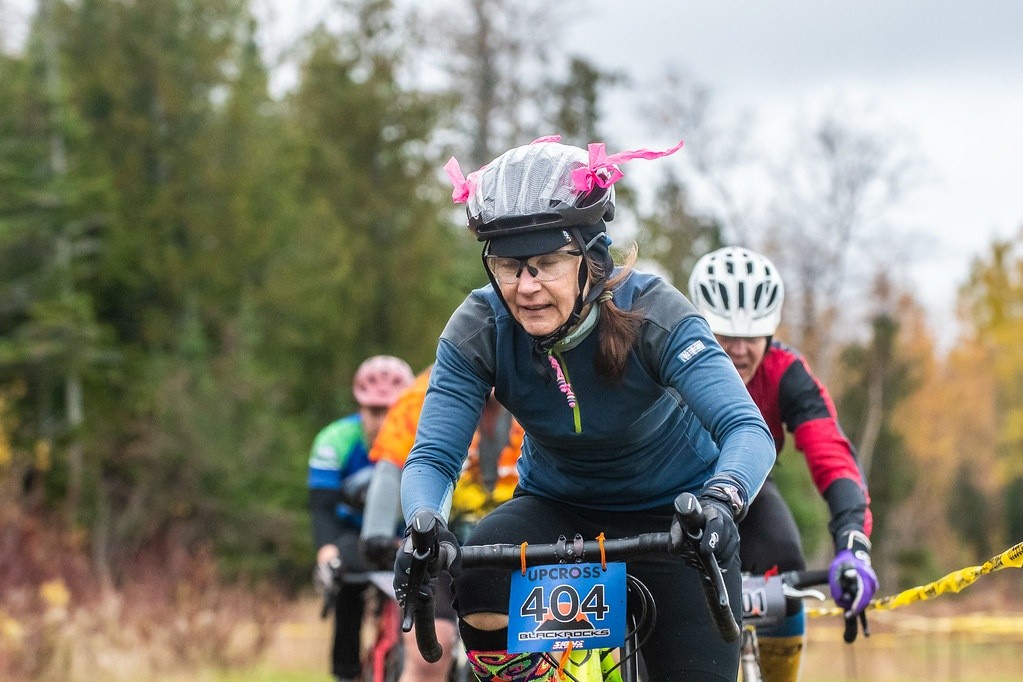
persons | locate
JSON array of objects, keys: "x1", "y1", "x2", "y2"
[
  {"x1": 690, "y1": 246, "x2": 880, "y2": 682},
  {"x1": 309, "y1": 352, "x2": 526, "y2": 682},
  {"x1": 398, "y1": 144, "x2": 777, "y2": 682}
]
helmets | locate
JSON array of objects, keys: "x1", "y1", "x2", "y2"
[
  {"x1": 352, "y1": 355, "x2": 414, "y2": 407},
  {"x1": 687, "y1": 247, "x2": 784, "y2": 337},
  {"x1": 467, "y1": 143, "x2": 617, "y2": 237}
]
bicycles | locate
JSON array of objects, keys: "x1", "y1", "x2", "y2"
[
  {"x1": 733, "y1": 563, "x2": 871, "y2": 682},
  {"x1": 319, "y1": 536, "x2": 403, "y2": 682},
  {"x1": 403, "y1": 492, "x2": 740, "y2": 682}
]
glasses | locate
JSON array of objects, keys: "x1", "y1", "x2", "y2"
[{"x1": 483, "y1": 232, "x2": 608, "y2": 285}]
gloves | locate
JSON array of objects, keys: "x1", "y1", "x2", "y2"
[
  {"x1": 394, "y1": 513, "x2": 461, "y2": 612},
  {"x1": 668, "y1": 481, "x2": 741, "y2": 565},
  {"x1": 829, "y1": 526, "x2": 879, "y2": 619}
]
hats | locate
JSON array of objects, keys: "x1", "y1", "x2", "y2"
[{"x1": 485, "y1": 217, "x2": 609, "y2": 257}]
